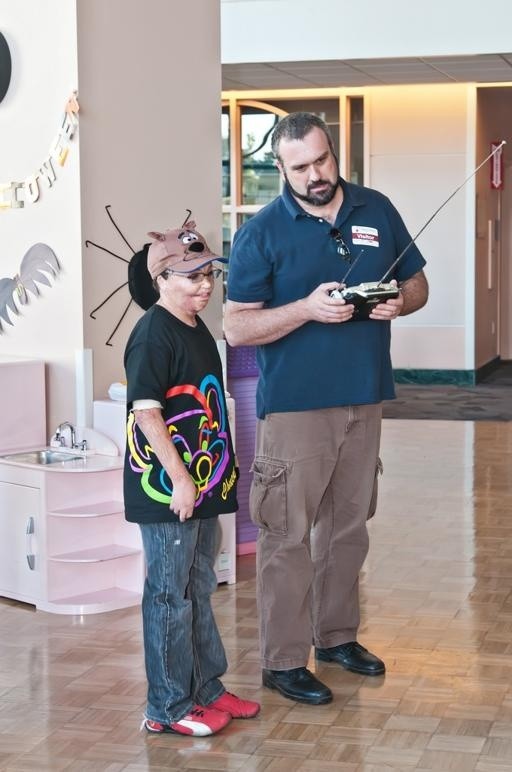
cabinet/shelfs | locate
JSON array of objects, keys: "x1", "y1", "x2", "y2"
[
  {"x1": 150, "y1": 394, "x2": 236, "y2": 586},
  {"x1": 1, "y1": 357, "x2": 47, "y2": 455},
  {"x1": 2, "y1": 456, "x2": 143, "y2": 616}
]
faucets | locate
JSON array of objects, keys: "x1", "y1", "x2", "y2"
[{"x1": 54, "y1": 421, "x2": 78, "y2": 449}]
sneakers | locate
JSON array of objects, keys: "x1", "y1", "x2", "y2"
[{"x1": 144, "y1": 689, "x2": 260, "y2": 737}]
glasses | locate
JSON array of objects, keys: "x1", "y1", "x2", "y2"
[
  {"x1": 167, "y1": 265, "x2": 222, "y2": 283},
  {"x1": 330, "y1": 228, "x2": 351, "y2": 257}
]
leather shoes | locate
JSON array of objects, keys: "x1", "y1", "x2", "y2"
[
  {"x1": 262, "y1": 668, "x2": 332, "y2": 704},
  {"x1": 316, "y1": 641, "x2": 384, "y2": 675}
]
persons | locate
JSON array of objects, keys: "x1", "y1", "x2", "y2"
[
  {"x1": 123, "y1": 222, "x2": 260, "y2": 736},
  {"x1": 222, "y1": 112, "x2": 429, "y2": 706}
]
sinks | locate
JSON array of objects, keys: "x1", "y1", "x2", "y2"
[{"x1": 1, "y1": 451, "x2": 90, "y2": 466}]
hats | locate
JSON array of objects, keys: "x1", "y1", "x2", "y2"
[{"x1": 147, "y1": 221, "x2": 228, "y2": 280}]
